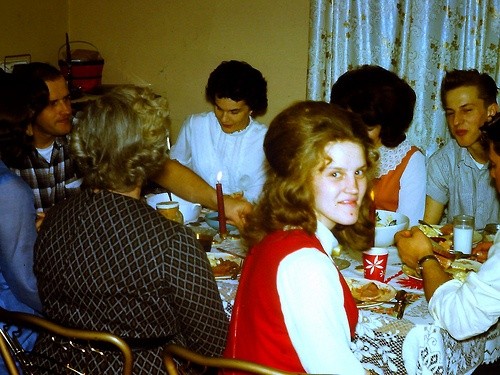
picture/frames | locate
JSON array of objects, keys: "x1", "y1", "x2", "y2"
[{"x1": 4, "y1": 54, "x2": 31, "y2": 73}]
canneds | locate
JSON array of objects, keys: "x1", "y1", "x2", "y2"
[{"x1": 156, "y1": 202, "x2": 184, "y2": 225}]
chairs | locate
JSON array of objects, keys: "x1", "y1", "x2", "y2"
[{"x1": 0, "y1": 306, "x2": 133, "y2": 375}]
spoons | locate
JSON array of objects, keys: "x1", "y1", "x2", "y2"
[{"x1": 395, "y1": 289, "x2": 407, "y2": 320}]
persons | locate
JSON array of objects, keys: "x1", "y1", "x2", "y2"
[
  {"x1": 171, "y1": 60, "x2": 270, "y2": 205},
  {"x1": 214, "y1": 99, "x2": 370, "y2": 375},
  {"x1": 0, "y1": 67, "x2": 51, "y2": 375},
  {"x1": 426, "y1": 68, "x2": 500, "y2": 227},
  {"x1": 31, "y1": 86, "x2": 230, "y2": 375},
  {"x1": 8, "y1": 61, "x2": 252, "y2": 231},
  {"x1": 394, "y1": 113, "x2": 500, "y2": 341},
  {"x1": 331, "y1": 64, "x2": 426, "y2": 230}
]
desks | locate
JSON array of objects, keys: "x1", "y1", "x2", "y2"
[{"x1": 68, "y1": 83, "x2": 119, "y2": 119}]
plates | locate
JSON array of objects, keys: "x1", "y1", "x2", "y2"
[
  {"x1": 344, "y1": 277, "x2": 396, "y2": 308},
  {"x1": 207, "y1": 252, "x2": 244, "y2": 278},
  {"x1": 406, "y1": 224, "x2": 495, "y2": 281}
]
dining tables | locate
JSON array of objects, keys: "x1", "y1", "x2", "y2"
[{"x1": 195, "y1": 218, "x2": 500, "y2": 375}]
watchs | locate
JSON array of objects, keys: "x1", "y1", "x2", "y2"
[{"x1": 415, "y1": 254, "x2": 438, "y2": 277}]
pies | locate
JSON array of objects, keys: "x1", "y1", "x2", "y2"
[
  {"x1": 352, "y1": 282, "x2": 381, "y2": 302},
  {"x1": 212, "y1": 258, "x2": 239, "y2": 277}
]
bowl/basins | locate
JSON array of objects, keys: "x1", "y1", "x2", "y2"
[
  {"x1": 374, "y1": 210, "x2": 409, "y2": 247},
  {"x1": 146, "y1": 193, "x2": 202, "y2": 224},
  {"x1": 205, "y1": 212, "x2": 239, "y2": 232}
]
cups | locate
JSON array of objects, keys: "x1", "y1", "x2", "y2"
[
  {"x1": 453, "y1": 214, "x2": 474, "y2": 255},
  {"x1": 363, "y1": 247, "x2": 390, "y2": 283}
]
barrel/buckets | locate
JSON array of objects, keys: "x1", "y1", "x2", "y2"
[{"x1": 58, "y1": 41, "x2": 104, "y2": 92}]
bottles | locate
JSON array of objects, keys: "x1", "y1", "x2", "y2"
[{"x1": 63, "y1": 30, "x2": 81, "y2": 99}]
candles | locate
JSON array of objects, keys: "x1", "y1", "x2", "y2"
[{"x1": 216, "y1": 172, "x2": 226, "y2": 234}]
